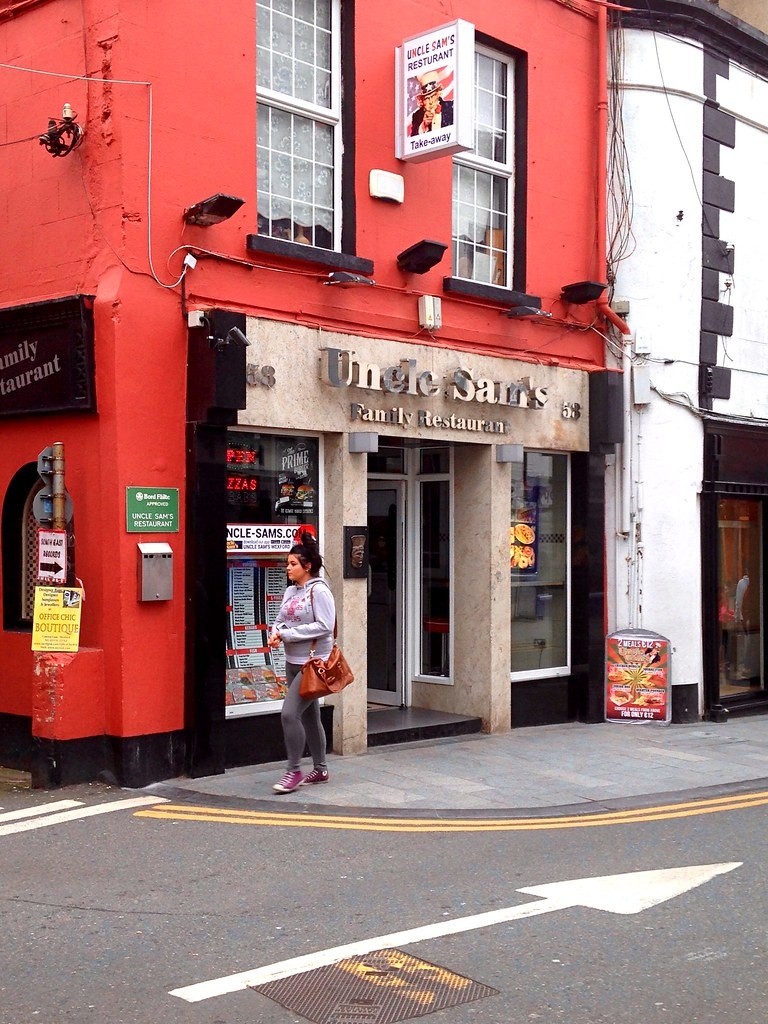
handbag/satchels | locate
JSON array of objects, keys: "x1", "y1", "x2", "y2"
[{"x1": 299, "y1": 644, "x2": 355, "y2": 700}]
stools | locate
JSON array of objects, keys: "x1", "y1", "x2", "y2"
[{"x1": 423, "y1": 617, "x2": 449, "y2": 676}]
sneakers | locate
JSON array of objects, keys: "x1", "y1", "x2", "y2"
[
  {"x1": 271, "y1": 769, "x2": 306, "y2": 792},
  {"x1": 300, "y1": 769, "x2": 329, "y2": 786}
]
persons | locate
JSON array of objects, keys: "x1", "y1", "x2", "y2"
[
  {"x1": 268, "y1": 533, "x2": 336, "y2": 792},
  {"x1": 734, "y1": 562, "x2": 755, "y2": 681}
]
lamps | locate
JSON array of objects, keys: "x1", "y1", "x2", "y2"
[
  {"x1": 499, "y1": 304, "x2": 552, "y2": 319},
  {"x1": 395, "y1": 240, "x2": 448, "y2": 274},
  {"x1": 324, "y1": 270, "x2": 376, "y2": 288},
  {"x1": 183, "y1": 192, "x2": 245, "y2": 225},
  {"x1": 558, "y1": 279, "x2": 608, "y2": 304}
]
